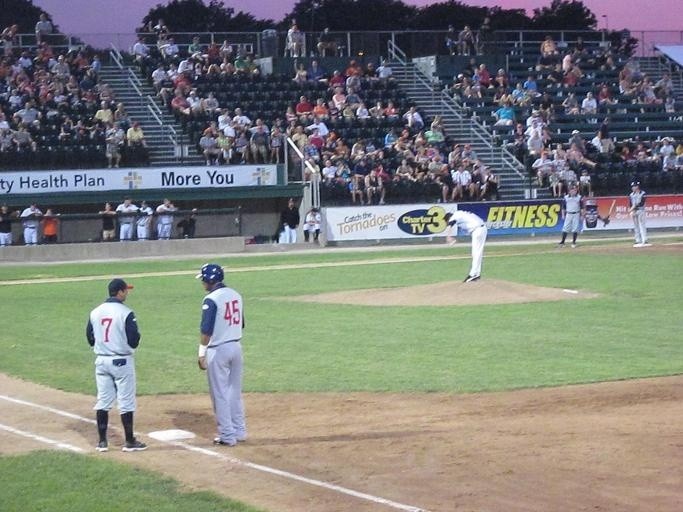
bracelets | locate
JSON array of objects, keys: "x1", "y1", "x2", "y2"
[{"x1": 196, "y1": 344, "x2": 207, "y2": 358}]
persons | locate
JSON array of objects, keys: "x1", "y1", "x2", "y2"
[
  {"x1": 85, "y1": 277, "x2": 148, "y2": 451},
  {"x1": 444, "y1": 20, "x2": 683, "y2": 196},
  {"x1": 628, "y1": 182, "x2": 650, "y2": 245},
  {"x1": 0, "y1": 202, "x2": 58, "y2": 245},
  {"x1": 99, "y1": 198, "x2": 197, "y2": 240},
  {"x1": 193, "y1": 264, "x2": 248, "y2": 447},
  {"x1": 133, "y1": 18, "x2": 499, "y2": 206},
  {"x1": 0, "y1": 13, "x2": 145, "y2": 168},
  {"x1": 277, "y1": 198, "x2": 322, "y2": 245},
  {"x1": 555, "y1": 185, "x2": 585, "y2": 249},
  {"x1": 441, "y1": 210, "x2": 487, "y2": 283}
]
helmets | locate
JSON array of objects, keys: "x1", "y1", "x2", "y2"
[
  {"x1": 200, "y1": 263, "x2": 223, "y2": 285},
  {"x1": 630, "y1": 181, "x2": 640, "y2": 187}
]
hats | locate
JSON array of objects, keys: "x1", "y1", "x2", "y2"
[
  {"x1": 571, "y1": 130, "x2": 579, "y2": 135},
  {"x1": 108, "y1": 279, "x2": 133, "y2": 292}
]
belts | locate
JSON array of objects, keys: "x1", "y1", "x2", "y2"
[{"x1": 566, "y1": 211, "x2": 580, "y2": 214}]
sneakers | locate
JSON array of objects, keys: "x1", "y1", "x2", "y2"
[
  {"x1": 121, "y1": 437, "x2": 148, "y2": 452},
  {"x1": 94, "y1": 440, "x2": 108, "y2": 451},
  {"x1": 463, "y1": 274, "x2": 480, "y2": 282},
  {"x1": 212, "y1": 437, "x2": 235, "y2": 447},
  {"x1": 552, "y1": 243, "x2": 576, "y2": 249}
]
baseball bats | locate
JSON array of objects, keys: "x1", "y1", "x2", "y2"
[
  {"x1": 604, "y1": 199, "x2": 617, "y2": 227},
  {"x1": 627, "y1": 205, "x2": 636, "y2": 212}
]
